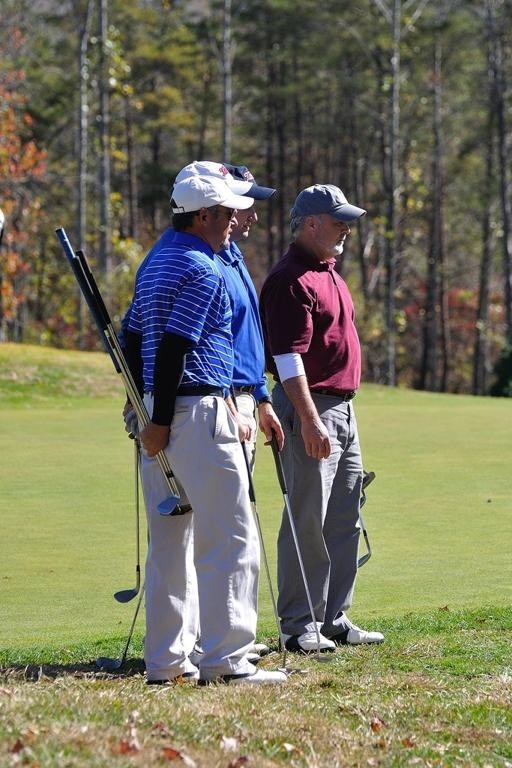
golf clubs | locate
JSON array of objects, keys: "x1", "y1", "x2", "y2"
[
  {"x1": 97, "y1": 580, "x2": 146, "y2": 674},
  {"x1": 55, "y1": 228, "x2": 195, "y2": 518},
  {"x1": 270, "y1": 437, "x2": 334, "y2": 662},
  {"x1": 114, "y1": 442, "x2": 142, "y2": 604},
  {"x1": 242, "y1": 440, "x2": 302, "y2": 675},
  {"x1": 358, "y1": 473, "x2": 376, "y2": 567}
]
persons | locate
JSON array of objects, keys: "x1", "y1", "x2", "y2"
[
  {"x1": 257, "y1": 182, "x2": 386, "y2": 655},
  {"x1": 123, "y1": 172, "x2": 292, "y2": 688},
  {"x1": 117, "y1": 159, "x2": 272, "y2": 666},
  {"x1": 214, "y1": 161, "x2": 286, "y2": 487}
]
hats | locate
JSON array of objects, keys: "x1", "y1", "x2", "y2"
[
  {"x1": 169, "y1": 158, "x2": 277, "y2": 214},
  {"x1": 290, "y1": 184, "x2": 367, "y2": 223}
]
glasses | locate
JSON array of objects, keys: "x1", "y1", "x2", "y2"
[{"x1": 227, "y1": 209, "x2": 238, "y2": 218}]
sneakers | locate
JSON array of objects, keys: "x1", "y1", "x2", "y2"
[
  {"x1": 145, "y1": 665, "x2": 203, "y2": 687},
  {"x1": 244, "y1": 650, "x2": 262, "y2": 664},
  {"x1": 323, "y1": 622, "x2": 385, "y2": 646},
  {"x1": 196, "y1": 660, "x2": 289, "y2": 686},
  {"x1": 277, "y1": 629, "x2": 338, "y2": 654},
  {"x1": 249, "y1": 641, "x2": 270, "y2": 655}
]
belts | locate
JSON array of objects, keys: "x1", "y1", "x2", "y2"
[
  {"x1": 310, "y1": 386, "x2": 358, "y2": 402},
  {"x1": 176, "y1": 382, "x2": 225, "y2": 398},
  {"x1": 229, "y1": 382, "x2": 258, "y2": 395}
]
